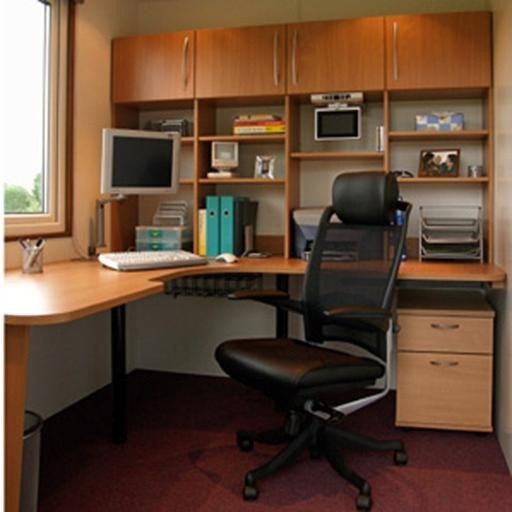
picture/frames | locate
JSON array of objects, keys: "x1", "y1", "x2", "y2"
[{"x1": 417, "y1": 146, "x2": 463, "y2": 177}]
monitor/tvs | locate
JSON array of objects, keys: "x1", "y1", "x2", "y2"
[
  {"x1": 313, "y1": 103, "x2": 362, "y2": 142},
  {"x1": 99, "y1": 127, "x2": 181, "y2": 194}
]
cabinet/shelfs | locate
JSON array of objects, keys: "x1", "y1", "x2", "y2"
[
  {"x1": 393, "y1": 289, "x2": 497, "y2": 441},
  {"x1": 194, "y1": 13, "x2": 386, "y2": 106},
  {"x1": 111, "y1": 27, "x2": 196, "y2": 110},
  {"x1": 285, "y1": 92, "x2": 390, "y2": 262},
  {"x1": 384, "y1": 10, "x2": 494, "y2": 100},
  {"x1": 384, "y1": 90, "x2": 496, "y2": 262},
  {"x1": 195, "y1": 95, "x2": 300, "y2": 261},
  {"x1": 104, "y1": 105, "x2": 216, "y2": 253},
  {"x1": 134, "y1": 224, "x2": 192, "y2": 251}
]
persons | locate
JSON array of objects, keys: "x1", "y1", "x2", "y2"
[{"x1": 423, "y1": 152, "x2": 458, "y2": 176}]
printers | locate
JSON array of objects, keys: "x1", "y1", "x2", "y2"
[{"x1": 292, "y1": 206, "x2": 386, "y2": 261}]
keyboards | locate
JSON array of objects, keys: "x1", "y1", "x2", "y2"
[{"x1": 97, "y1": 249, "x2": 207, "y2": 271}]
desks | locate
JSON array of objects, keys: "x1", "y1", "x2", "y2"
[{"x1": 4, "y1": 255, "x2": 509, "y2": 512}]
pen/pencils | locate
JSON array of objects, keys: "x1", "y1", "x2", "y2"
[{"x1": 18, "y1": 237, "x2": 46, "y2": 273}]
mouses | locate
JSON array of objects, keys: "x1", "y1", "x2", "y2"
[{"x1": 214, "y1": 253, "x2": 239, "y2": 263}]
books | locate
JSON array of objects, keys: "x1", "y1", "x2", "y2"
[{"x1": 232, "y1": 113, "x2": 286, "y2": 136}]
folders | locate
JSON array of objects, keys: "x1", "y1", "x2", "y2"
[{"x1": 206, "y1": 196, "x2": 249, "y2": 256}]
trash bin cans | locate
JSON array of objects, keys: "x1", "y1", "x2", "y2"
[{"x1": 20, "y1": 410, "x2": 44, "y2": 512}]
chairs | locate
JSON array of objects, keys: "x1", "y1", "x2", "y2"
[{"x1": 215, "y1": 169, "x2": 412, "y2": 512}]
